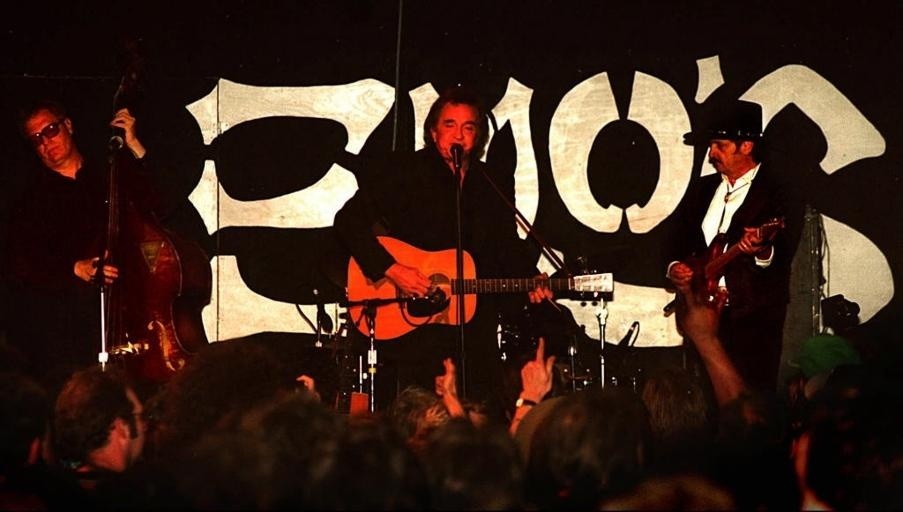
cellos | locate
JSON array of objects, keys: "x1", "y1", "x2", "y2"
[{"x1": 82, "y1": 68, "x2": 213, "y2": 412}]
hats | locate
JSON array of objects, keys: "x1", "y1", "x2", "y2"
[{"x1": 683, "y1": 99, "x2": 773, "y2": 152}]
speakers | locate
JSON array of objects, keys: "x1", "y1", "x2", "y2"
[{"x1": 773, "y1": 205, "x2": 820, "y2": 400}]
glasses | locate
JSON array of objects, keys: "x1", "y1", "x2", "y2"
[{"x1": 28, "y1": 119, "x2": 66, "y2": 147}]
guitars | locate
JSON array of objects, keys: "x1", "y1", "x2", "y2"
[
  {"x1": 676, "y1": 218, "x2": 786, "y2": 338},
  {"x1": 346, "y1": 236, "x2": 613, "y2": 341}
]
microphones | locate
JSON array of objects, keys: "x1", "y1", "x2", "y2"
[
  {"x1": 607, "y1": 323, "x2": 636, "y2": 371},
  {"x1": 107, "y1": 136, "x2": 124, "y2": 164},
  {"x1": 432, "y1": 289, "x2": 446, "y2": 304},
  {"x1": 450, "y1": 143, "x2": 463, "y2": 176},
  {"x1": 311, "y1": 283, "x2": 332, "y2": 333}
]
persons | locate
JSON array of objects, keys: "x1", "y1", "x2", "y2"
[
  {"x1": 662, "y1": 101, "x2": 810, "y2": 385},
  {"x1": 14, "y1": 103, "x2": 167, "y2": 402},
  {"x1": 340, "y1": 89, "x2": 554, "y2": 400}
]
